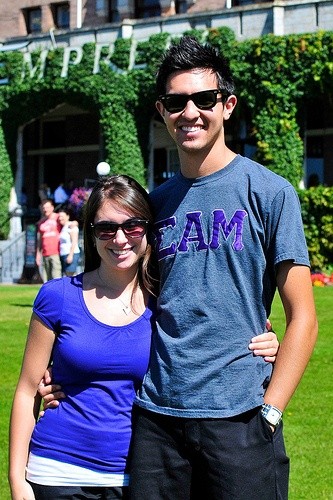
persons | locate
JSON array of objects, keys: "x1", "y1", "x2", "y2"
[
  {"x1": 128, "y1": 35, "x2": 318, "y2": 500},
  {"x1": 58, "y1": 210, "x2": 80, "y2": 277},
  {"x1": 54, "y1": 182, "x2": 68, "y2": 205},
  {"x1": 36, "y1": 199, "x2": 63, "y2": 283},
  {"x1": 9, "y1": 175, "x2": 279, "y2": 500}
]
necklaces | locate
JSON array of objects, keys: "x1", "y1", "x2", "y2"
[{"x1": 97, "y1": 270, "x2": 137, "y2": 316}]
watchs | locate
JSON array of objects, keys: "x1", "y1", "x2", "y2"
[{"x1": 261, "y1": 403, "x2": 282, "y2": 425}]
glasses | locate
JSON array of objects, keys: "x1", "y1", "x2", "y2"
[
  {"x1": 90, "y1": 218, "x2": 150, "y2": 240},
  {"x1": 158, "y1": 89, "x2": 228, "y2": 113}
]
